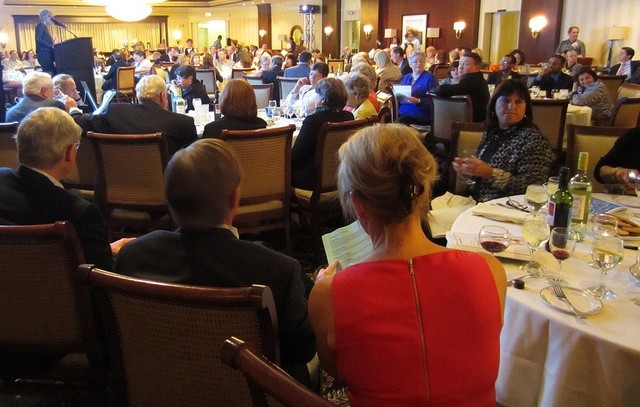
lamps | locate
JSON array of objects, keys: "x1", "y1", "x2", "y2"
[
  {"x1": 85, "y1": 1, "x2": 166, "y2": 23},
  {"x1": 385, "y1": 28, "x2": 397, "y2": 47},
  {"x1": 427, "y1": 27, "x2": 440, "y2": 46},
  {"x1": 453, "y1": 21, "x2": 466, "y2": 39},
  {"x1": 529, "y1": 16, "x2": 548, "y2": 38},
  {"x1": 606, "y1": 25, "x2": 624, "y2": 67}
]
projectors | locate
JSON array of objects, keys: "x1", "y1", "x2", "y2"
[{"x1": 299, "y1": 5, "x2": 320, "y2": 12}]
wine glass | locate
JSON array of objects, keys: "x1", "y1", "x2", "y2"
[
  {"x1": 633, "y1": 246, "x2": 639, "y2": 307},
  {"x1": 587, "y1": 230, "x2": 623, "y2": 301},
  {"x1": 477, "y1": 223, "x2": 510, "y2": 259},
  {"x1": 551, "y1": 88, "x2": 559, "y2": 98},
  {"x1": 591, "y1": 214, "x2": 617, "y2": 237},
  {"x1": 546, "y1": 174, "x2": 560, "y2": 201},
  {"x1": 529, "y1": 87, "x2": 537, "y2": 98},
  {"x1": 268, "y1": 99, "x2": 276, "y2": 120},
  {"x1": 538, "y1": 89, "x2": 547, "y2": 99},
  {"x1": 294, "y1": 105, "x2": 305, "y2": 128},
  {"x1": 191, "y1": 96, "x2": 202, "y2": 114},
  {"x1": 545, "y1": 226, "x2": 577, "y2": 285},
  {"x1": 286, "y1": 103, "x2": 293, "y2": 123},
  {"x1": 519, "y1": 213, "x2": 549, "y2": 273},
  {"x1": 274, "y1": 107, "x2": 281, "y2": 125},
  {"x1": 459, "y1": 148, "x2": 479, "y2": 185},
  {"x1": 540, "y1": 62, "x2": 553, "y2": 74},
  {"x1": 522, "y1": 182, "x2": 547, "y2": 219},
  {"x1": 280, "y1": 97, "x2": 289, "y2": 118},
  {"x1": 531, "y1": 85, "x2": 541, "y2": 92},
  {"x1": 450, "y1": 67, "x2": 459, "y2": 85}
]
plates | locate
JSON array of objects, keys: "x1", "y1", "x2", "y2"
[
  {"x1": 628, "y1": 263, "x2": 640, "y2": 284},
  {"x1": 613, "y1": 194, "x2": 640, "y2": 208},
  {"x1": 539, "y1": 285, "x2": 606, "y2": 318}
]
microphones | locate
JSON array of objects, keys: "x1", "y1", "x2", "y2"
[{"x1": 50, "y1": 17, "x2": 68, "y2": 29}]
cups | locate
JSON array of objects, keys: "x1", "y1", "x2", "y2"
[
  {"x1": 560, "y1": 87, "x2": 570, "y2": 100},
  {"x1": 52, "y1": 87, "x2": 66, "y2": 104}
]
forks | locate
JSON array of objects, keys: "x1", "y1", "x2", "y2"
[
  {"x1": 551, "y1": 282, "x2": 586, "y2": 319},
  {"x1": 506, "y1": 263, "x2": 548, "y2": 285}
]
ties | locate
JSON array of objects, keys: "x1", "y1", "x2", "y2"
[{"x1": 616, "y1": 63, "x2": 625, "y2": 76}]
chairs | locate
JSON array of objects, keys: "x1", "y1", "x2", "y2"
[
  {"x1": 85, "y1": 130, "x2": 176, "y2": 245},
  {"x1": 428, "y1": 92, "x2": 474, "y2": 154},
  {"x1": 217, "y1": 122, "x2": 297, "y2": 255},
  {"x1": 446, "y1": 118, "x2": 487, "y2": 192},
  {"x1": 241, "y1": 73, "x2": 264, "y2": 84},
  {"x1": 250, "y1": 82, "x2": 274, "y2": 108},
  {"x1": 151, "y1": 64, "x2": 166, "y2": 81},
  {"x1": 577, "y1": 56, "x2": 594, "y2": 66},
  {"x1": 614, "y1": 81, "x2": 640, "y2": 105},
  {"x1": 385, "y1": 83, "x2": 399, "y2": 123},
  {"x1": 117, "y1": 65, "x2": 135, "y2": 102},
  {"x1": 74, "y1": 262, "x2": 281, "y2": 406},
  {"x1": 0, "y1": 220, "x2": 106, "y2": 407},
  {"x1": 609, "y1": 95, "x2": 640, "y2": 127},
  {"x1": 231, "y1": 67, "x2": 255, "y2": 79},
  {"x1": 289, "y1": 114, "x2": 381, "y2": 268},
  {"x1": 377, "y1": 105, "x2": 390, "y2": 122},
  {"x1": 194, "y1": 68, "x2": 216, "y2": 99},
  {"x1": 219, "y1": 335, "x2": 349, "y2": 406},
  {"x1": 275, "y1": 75, "x2": 302, "y2": 107},
  {"x1": 528, "y1": 98, "x2": 570, "y2": 154},
  {"x1": 327, "y1": 59, "x2": 345, "y2": 72},
  {"x1": 480, "y1": 69, "x2": 493, "y2": 82},
  {"x1": 596, "y1": 74, "x2": 628, "y2": 102},
  {"x1": 0, "y1": 120, "x2": 19, "y2": 170},
  {"x1": 433, "y1": 64, "x2": 450, "y2": 80},
  {"x1": 565, "y1": 122, "x2": 634, "y2": 192}
]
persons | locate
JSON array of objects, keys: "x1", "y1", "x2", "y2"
[
  {"x1": 308, "y1": 124, "x2": 507, "y2": 407},
  {"x1": 292, "y1": 79, "x2": 355, "y2": 190},
  {"x1": 115, "y1": 137, "x2": 314, "y2": 389},
  {"x1": 566, "y1": 68, "x2": 615, "y2": 126},
  {"x1": 429, "y1": 50, "x2": 453, "y2": 80},
  {"x1": 401, "y1": 32, "x2": 414, "y2": 51},
  {"x1": 227, "y1": 35, "x2": 270, "y2": 69},
  {"x1": 5, "y1": 71, "x2": 77, "y2": 135},
  {"x1": 262, "y1": 55, "x2": 284, "y2": 88},
  {"x1": 340, "y1": 73, "x2": 378, "y2": 120},
  {"x1": 510, "y1": 50, "x2": 530, "y2": 75},
  {"x1": 92, "y1": 47, "x2": 153, "y2": 92},
  {"x1": 166, "y1": 66, "x2": 210, "y2": 113},
  {"x1": 426, "y1": 47, "x2": 435, "y2": 64},
  {"x1": 354, "y1": 52, "x2": 368, "y2": 61},
  {"x1": 343, "y1": 63, "x2": 380, "y2": 113},
  {"x1": 409, "y1": 29, "x2": 420, "y2": 51},
  {"x1": 374, "y1": 51, "x2": 402, "y2": 93},
  {"x1": 454, "y1": 78, "x2": 550, "y2": 202},
  {"x1": 554, "y1": 26, "x2": 585, "y2": 56},
  {"x1": 425, "y1": 52, "x2": 490, "y2": 155},
  {"x1": 202, "y1": 78, "x2": 267, "y2": 139},
  {"x1": 0, "y1": 49, "x2": 39, "y2": 70},
  {"x1": 375, "y1": 38, "x2": 384, "y2": 49},
  {"x1": 594, "y1": 115, "x2": 639, "y2": 186},
  {"x1": 353, "y1": 58, "x2": 369, "y2": 65},
  {"x1": 152, "y1": 35, "x2": 228, "y2": 81},
  {"x1": 1, "y1": 107, "x2": 137, "y2": 272},
  {"x1": 397, "y1": 51, "x2": 436, "y2": 127},
  {"x1": 286, "y1": 63, "x2": 329, "y2": 118},
  {"x1": 565, "y1": 51, "x2": 582, "y2": 75},
  {"x1": 290, "y1": 36, "x2": 295, "y2": 52},
  {"x1": 53, "y1": 72, "x2": 84, "y2": 107},
  {"x1": 283, "y1": 49, "x2": 325, "y2": 77},
  {"x1": 529, "y1": 55, "x2": 572, "y2": 98},
  {"x1": 611, "y1": 47, "x2": 640, "y2": 83},
  {"x1": 390, "y1": 47, "x2": 412, "y2": 74},
  {"x1": 35, "y1": 10, "x2": 56, "y2": 74},
  {"x1": 70, "y1": 75, "x2": 198, "y2": 220},
  {"x1": 450, "y1": 46, "x2": 488, "y2": 67},
  {"x1": 485, "y1": 56, "x2": 521, "y2": 85}
]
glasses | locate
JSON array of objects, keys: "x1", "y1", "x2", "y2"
[
  {"x1": 500, "y1": 61, "x2": 512, "y2": 64},
  {"x1": 74, "y1": 139, "x2": 80, "y2": 150},
  {"x1": 506, "y1": 196, "x2": 531, "y2": 212}
]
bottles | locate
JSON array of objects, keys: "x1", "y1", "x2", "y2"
[
  {"x1": 173, "y1": 85, "x2": 185, "y2": 114},
  {"x1": 213, "y1": 89, "x2": 222, "y2": 120},
  {"x1": 568, "y1": 151, "x2": 592, "y2": 242},
  {"x1": 336, "y1": 63, "x2": 342, "y2": 77},
  {"x1": 545, "y1": 165, "x2": 573, "y2": 251},
  {"x1": 329, "y1": 66, "x2": 334, "y2": 76}
]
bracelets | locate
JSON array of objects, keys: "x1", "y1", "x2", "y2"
[
  {"x1": 613, "y1": 168, "x2": 619, "y2": 187},
  {"x1": 75, "y1": 97, "x2": 82, "y2": 103}
]
